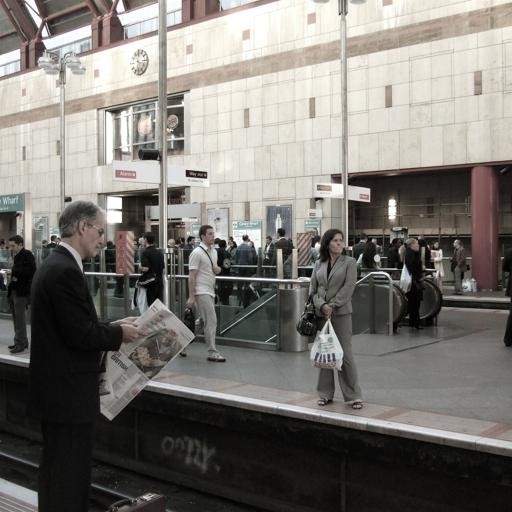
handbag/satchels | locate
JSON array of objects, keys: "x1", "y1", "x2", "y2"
[
  {"x1": 296, "y1": 292, "x2": 318, "y2": 336},
  {"x1": 400, "y1": 264, "x2": 412, "y2": 294}
]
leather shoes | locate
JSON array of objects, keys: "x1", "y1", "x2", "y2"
[
  {"x1": 8, "y1": 342, "x2": 28, "y2": 353},
  {"x1": 415, "y1": 324, "x2": 424, "y2": 329}
]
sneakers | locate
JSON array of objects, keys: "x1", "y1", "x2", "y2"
[
  {"x1": 207, "y1": 351, "x2": 226, "y2": 362},
  {"x1": 180, "y1": 350, "x2": 186, "y2": 357}
]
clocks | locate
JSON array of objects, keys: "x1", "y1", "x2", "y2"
[{"x1": 129, "y1": 49, "x2": 149, "y2": 75}]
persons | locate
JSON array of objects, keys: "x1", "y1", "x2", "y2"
[
  {"x1": 502, "y1": 247, "x2": 512, "y2": 347},
  {"x1": 3, "y1": 235, "x2": 37, "y2": 353},
  {"x1": 180, "y1": 225, "x2": 226, "y2": 362},
  {"x1": 393, "y1": 238, "x2": 424, "y2": 334},
  {"x1": 308, "y1": 229, "x2": 364, "y2": 409},
  {"x1": 450, "y1": 239, "x2": 467, "y2": 295},
  {"x1": 29, "y1": 200, "x2": 148, "y2": 511}
]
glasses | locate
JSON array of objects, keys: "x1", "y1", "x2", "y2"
[{"x1": 87, "y1": 223, "x2": 104, "y2": 237}]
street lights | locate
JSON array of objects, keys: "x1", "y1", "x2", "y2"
[{"x1": 36, "y1": 49, "x2": 86, "y2": 214}]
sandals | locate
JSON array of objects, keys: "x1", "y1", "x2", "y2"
[
  {"x1": 352, "y1": 401, "x2": 363, "y2": 409},
  {"x1": 318, "y1": 397, "x2": 332, "y2": 406}
]
